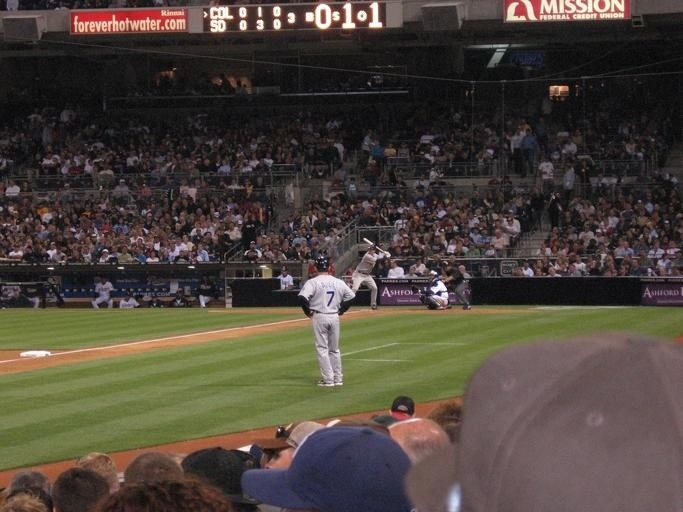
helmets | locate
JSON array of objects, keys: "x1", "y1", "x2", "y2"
[
  {"x1": 313, "y1": 255, "x2": 330, "y2": 271},
  {"x1": 426, "y1": 269, "x2": 438, "y2": 278},
  {"x1": 366, "y1": 245, "x2": 376, "y2": 251}
]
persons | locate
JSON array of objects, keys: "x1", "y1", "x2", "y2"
[
  {"x1": 0, "y1": 53, "x2": 683, "y2": 305},
  {"x1": 1, "y1": 330, "x2": 683, "y2": 512},
  {"x1": 297, "y1": 256, "x2": 356, "y2": 386},
  {"x1": 0, "y1": 0, "x2": 282, "y2": 11}
]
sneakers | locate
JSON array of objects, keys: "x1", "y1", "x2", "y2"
[
  {"x1": 317, "y1": 379, "x2": 344, "y2": 386},
  {"x1": 369, "y1": 302, "x2": 377, "y2": 310}
]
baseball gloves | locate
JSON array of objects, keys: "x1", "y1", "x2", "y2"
[{"x1": 412, "y1": 286, "x2": 419, "y2": 294}]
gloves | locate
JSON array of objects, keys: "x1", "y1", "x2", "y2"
[
  {"x1": 337, "y1": 306, "x2": 346, "y2": 316},
  {"x1": 304, "y1": 309, "x2": 315, "y2": 318}
]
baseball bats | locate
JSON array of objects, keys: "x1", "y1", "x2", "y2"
[{"x1": 364, "y1": 238, "x2": 384, "y2": 253}]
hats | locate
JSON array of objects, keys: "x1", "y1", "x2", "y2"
[
  {"x1": 390, "y1": 395, "x2": 416, "y2": 419},
  {"x1": 179, "y1": 446, "x2": 261, "y2": 506},
  {"x1": 400, "y1": 330, "x2": 683, "y2": 511},
  {"x1": 239, "y1": 428, "x2": 416, "y2": 512},
  {"x1": 251, "y1": 420, "x2": 325, "y2": 452}
]
